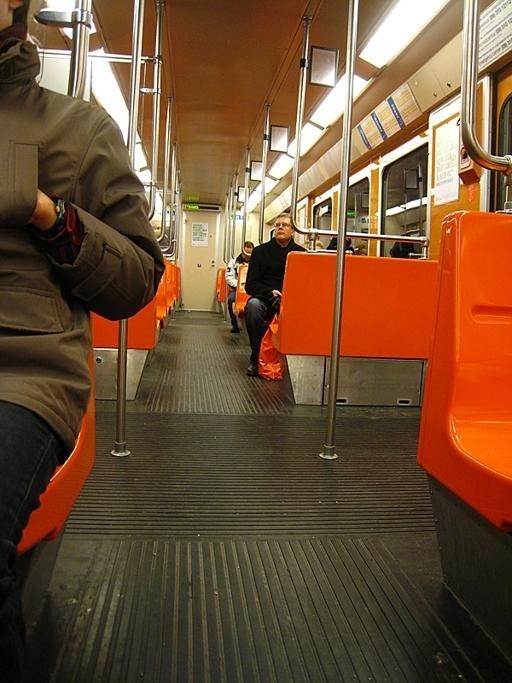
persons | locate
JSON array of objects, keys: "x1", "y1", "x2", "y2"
[
  {"x1": 225, "y1": 242, "x2": 254, "y2": 331},
  {"x1": 244, "y1": 213, "x2": 307, "y2": 376},
  {"x1": 2, "y1": 2, "x2": 166, "y2": 681},
  {"x1": 326, "y1": 237, "x2": 355, "y2": 253}
]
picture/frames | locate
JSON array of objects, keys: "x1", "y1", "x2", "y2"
[
  {"x1": 268, "y1": 123, "x2": 290, "y2": 154},
  {"x1": 237, "y1": 186, "x2": 250, "y2": 204},
  {"x1": 250, "y1": 159, "x2": 262, "y2": 182},
  {"x1": 309, "y1": 45, "x2": 341, "y2": 88}
]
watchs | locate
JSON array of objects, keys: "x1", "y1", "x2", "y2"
[{"x1": 42, "y1": 199, "x2": 76, "y2": 239}]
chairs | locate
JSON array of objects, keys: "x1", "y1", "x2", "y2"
[
  {"x1": 13, "y1": 257, "x2": 183, "y2": 554},
  {"x1": 417, "y1": 210, "x2": 512, "y2": 529},
  {"x1": 217, "y1": 249, "x2": 438, "y2": 406}
]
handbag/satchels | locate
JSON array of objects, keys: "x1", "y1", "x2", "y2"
[{"x1": 258, "y1": 313, "x2": 287, "y2": 381}]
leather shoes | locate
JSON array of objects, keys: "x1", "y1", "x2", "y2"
[{"x1": 247, "y1": 360, "x2": 257, "y2": 376}]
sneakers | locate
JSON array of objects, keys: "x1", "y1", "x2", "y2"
[{"x1": 231, "y1": 328, "x2": 239, "y2": 333}]
